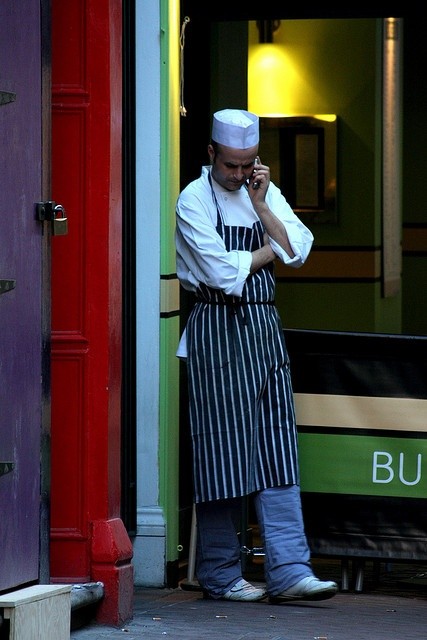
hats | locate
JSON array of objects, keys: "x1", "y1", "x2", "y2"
[{"x1": 211, "y1": 109, "x2": 260, "y2": 150}]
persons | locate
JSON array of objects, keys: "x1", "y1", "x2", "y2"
[{"x1": 173, "y1": 106, "x2": 340, "y2": 604}]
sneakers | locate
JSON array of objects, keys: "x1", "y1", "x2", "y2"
[
  {"x1": 203, "y1": 579, "x2": 268, "y2": 602},
  {"x1": 271, "y1": 576, "x2": 338, "y2": 602}
]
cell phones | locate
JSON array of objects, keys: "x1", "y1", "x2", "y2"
[{"x1": 252, "y1": 157, "x2": 261, "y2": 189}]
